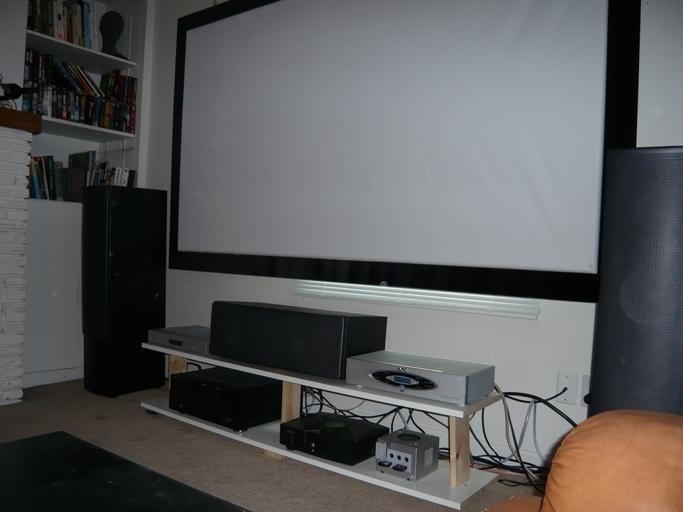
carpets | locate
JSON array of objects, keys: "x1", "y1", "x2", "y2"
[{"x1": 1, "y1": 429, "x2": 251, "y2": 512}]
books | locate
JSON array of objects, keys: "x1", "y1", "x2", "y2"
[{"x1": 22, "y1": 0, "x2": 139, "y2": 203}]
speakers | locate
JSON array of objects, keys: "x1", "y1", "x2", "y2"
[
  {"x1": 208, "y1": 299, "x2": 388, "y2": 381},
  {"x1": 586, "y1": 144, "x2": 682, "y2": 421},
  {"x1": 80, "y1": 184, "x2": 168, "y2": 398}
]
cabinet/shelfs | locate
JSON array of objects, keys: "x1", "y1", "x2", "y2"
[
  {"x1": 18, "y1": 1, "x2": 155, "y2": 388},
  {"x1": 138, "y1": 335, "x2": 505, "y2": 512}
]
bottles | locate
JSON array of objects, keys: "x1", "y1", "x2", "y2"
[{"x1": 0, "y1": 82, "x2": 40, "y2": 101}]
